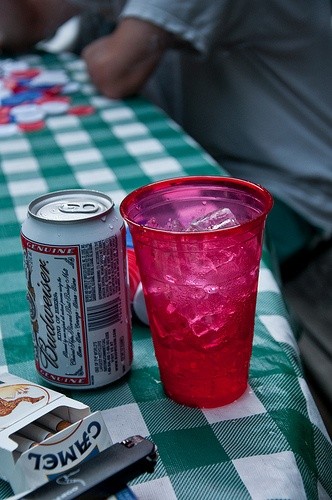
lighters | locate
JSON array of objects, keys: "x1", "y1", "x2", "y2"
[{"x1": 16, "y1": 434, "x2": 158, "y2": 500}]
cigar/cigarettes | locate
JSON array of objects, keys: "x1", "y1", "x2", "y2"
[
  {"x1": 9, "y1": 435, "x2": 41, "y2": 452},
  {"x1": 18, "y1": 424, "x2": 58, "y2": 442},
  {"x1": 39, "y1": 415, "x2": 73, "y2": 431}
]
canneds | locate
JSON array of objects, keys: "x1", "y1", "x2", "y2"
[{"x1": 20, "y1": 189, "x2": 133, "y2": 390}]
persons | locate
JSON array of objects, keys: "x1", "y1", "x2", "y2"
[{"x1": 0, "y1": 0, "x2": 332, "y2": 401}]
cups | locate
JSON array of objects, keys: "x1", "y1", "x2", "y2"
[{"x1": 120, "y1": 177, "x2": 273, "y2": 409}]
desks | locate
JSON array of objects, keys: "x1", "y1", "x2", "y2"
[{"x1": 1, "y1": 49, "x2": 332, "y2": 500}]
